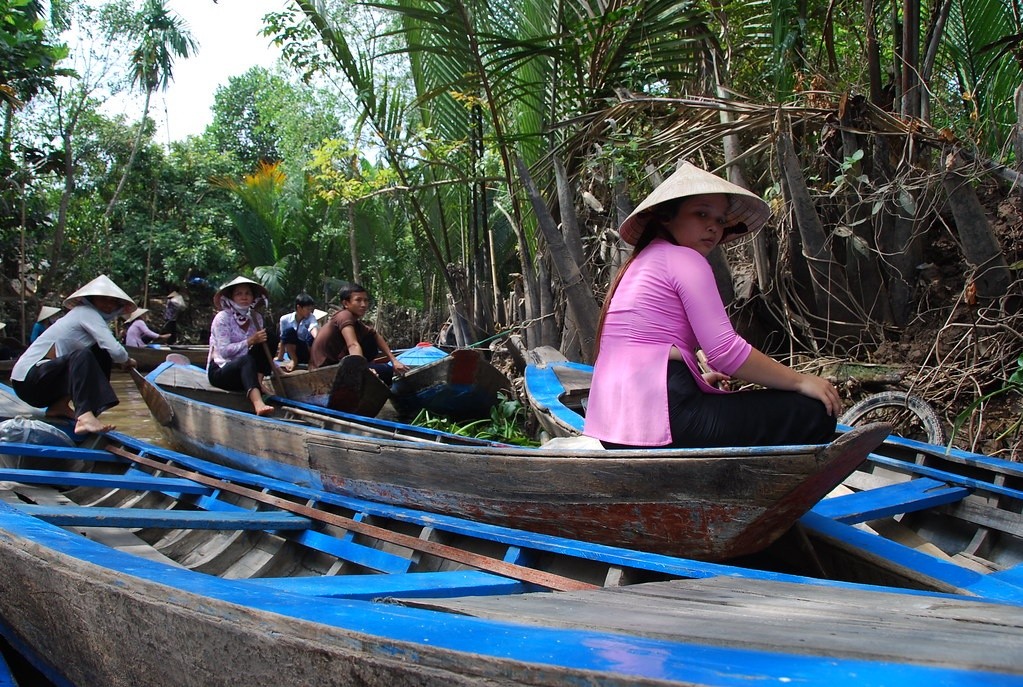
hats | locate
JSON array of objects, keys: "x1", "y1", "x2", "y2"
[
  {"x1": 37, "y1": 306, "x2": 62, "y2": 322},
  {"x1": 167, "y1": 291, "x2": 179, "y2": 298},
  {"x1": 617, "y1": 162, "x2": 771, "y2": 246},
  {"x1": 124, "y1": 307, "x2": 149, "y2": 323},
  {"x1": 213, "y1": 276, "x2": 268, "y2": 311},
  {"x1": 312, "y1": 308, "x2": 328, "y2": 321},
  {"x1": 171, "y1": 295, "x2": 187, "y2": 308},
  {"x1": 62, "y1": 273, "x2": 137, "y2": 309},
  {"x1": 0, "y1": 323, "x2": 6, "y2": 330}
]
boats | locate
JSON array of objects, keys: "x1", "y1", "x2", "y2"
[
  {"x1": 387, "y1": 342, "x2": 513, "y2": 425},
  {"x1": 262, "y1": 343, "x2": 395, "y2": 419},
  {"x1": 141, "y1": 354, "x2": 892, "y2": 568},
  {"x1": 123, "y1": 342, "x2": 210, "y2": 372},
  {"x1": 523, "y1": 361, "x2": 1023, "y2": 607},
  {"x1": 1, "y1": 383, "x2": 1023, "y2": 686}
]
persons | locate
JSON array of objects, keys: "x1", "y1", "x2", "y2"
[
  {"x1": 583, "y1": 163, "x2": 842, "y2": 450},
  {"x1": 124, "y1": 308, "x2": 171, "y2": 347},
  {"x1": 273, "y1": 293, "x2": 319, "y2": 371},
  {"x1": 309, "y1": 284, "x2": 410, "y2": 375},
  {"x1": 30, "y1": 306, "x2": 62, "y2": 342},
  {"x1": 206, "y1": 276, "x2": 277, "y2": 416},
  {"x1": 10, "y1": 274, "x2": 137, "y2": 435},
  {"x1": 164, "y1": 290, "x2": 178, "y2": 344}
]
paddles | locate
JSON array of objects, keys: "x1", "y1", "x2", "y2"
[
  {"x1": 281, "y1": 406, "x2": 449, "y2": 445},
  {"x1": 251, "y1": 309, "x2": 288, "y2": 399},
  {"x1": 127, "y1": 364, "x2": 174, "y2": 426}
]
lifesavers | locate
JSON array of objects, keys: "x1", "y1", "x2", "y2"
[{"x1": 836, "y1": 390, "x2": 947, "y2": 447}]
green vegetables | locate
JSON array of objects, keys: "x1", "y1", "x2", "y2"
[{"x1": 411, "y1": 391, "x2": 543, "y2": 447}]
